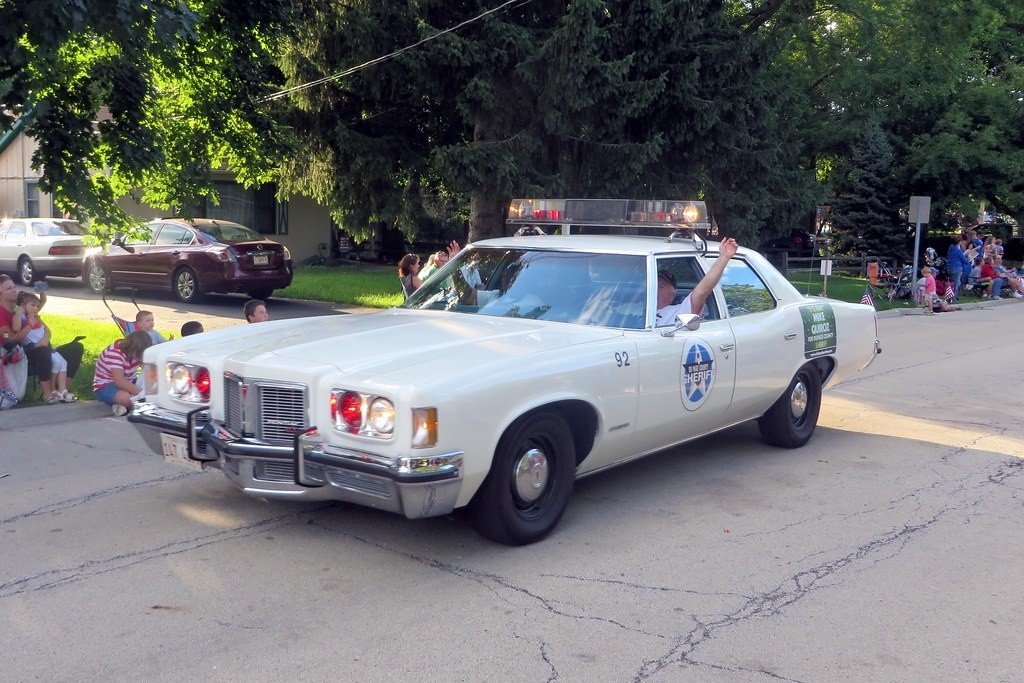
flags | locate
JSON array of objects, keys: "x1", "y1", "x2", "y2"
[
  {"x1": 946, "y1": 282, "x2": 954, "y2": 299},
  {"x1": 860, "y1": 289, "x2": 875, "y2": 306}
]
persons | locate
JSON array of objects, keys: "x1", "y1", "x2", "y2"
[
  {"x1": 655, "y1": 237, "x2": 738, "y2": 327},
  {"x1": 244, "y1": 301, "x2": 269, "y2": 323},
  {"x1": 136, "y1": 311, "x2": 166, "y2": 345},
  {"x1": 0, "y1": 274, "x2": 84, "y2": 404},
  {"x1": 181, "y1": 321, "x2": 204, "y2": 337},
  {"x1": 947, "y1": 230, "x2": 1024, "y2": 302},
  {"x1": 996, "y1": 216, "x2": 1005, "y2": 223},
  {"x1": 398, "y1": 240, "x2": 546, "y2": 318},
  {"x1": 916, "y1": 267, "x2": 939, "y2": 314},
  {"x1": 93, "y1": 331, "x2": 152, "y2": 416}
]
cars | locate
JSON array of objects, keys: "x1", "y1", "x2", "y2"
[
  {"x1": 0, "y1": 217, "x2": 101, "y2": 287},
  {"x1": 965, "y1": 221, "x2": 1013, "y2": 237},
  {"x1": 80, "y1": 216, "x2": 295, "y2": 305},
  {"x1": 126, "y1": 198, "x2": 884, "y2": 547}
]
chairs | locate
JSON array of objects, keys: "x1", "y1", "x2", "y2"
[
  {"x1": 25, "y1": 335, "x2": 87, "y2": 400},
  {"x1": 866, "y1": 262, "x2": 887, "y2": 300},
  {"x1": 961, "y1": 265, "x2": 1013, "y2": 298},
  {"x1": 889, "y1": 269, "x2": 912, "y2": 302}
]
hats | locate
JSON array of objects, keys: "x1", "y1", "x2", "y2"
[
  {"x1": 658, "y1": 269, "x2": 676, "y2": 289},
  {"x1": 930, "y1": 267, "x2": 939, "y2": 273}
]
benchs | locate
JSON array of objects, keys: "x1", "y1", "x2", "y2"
[{"x1": 575, "y1": 285, "x2": 691, "y2": 303}]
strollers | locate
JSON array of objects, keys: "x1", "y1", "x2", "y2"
[{"x1": 885, "y1": 264, "x2": 913, "y2": 300}]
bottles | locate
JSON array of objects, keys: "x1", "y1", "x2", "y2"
[{"x1": 3, "y1": 332, "x2": 9, "y2": 346}]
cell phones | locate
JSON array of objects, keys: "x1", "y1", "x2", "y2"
[{"x1": 34, "y1": 282, "x2": 49, "y2": 292}]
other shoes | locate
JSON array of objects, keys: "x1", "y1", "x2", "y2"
[
  {"x1": 112, "y1": 404, "x2": 128, "y2": 417},
  {"x1": 1011, "y1": 291, "x2": 1023, "y2": 298},
  {"x1": 992, "y1": 295, "x2": 1003, "y2": 300},
  {"x1": 924, "y1": 310, "x2": 933, "y2": 315}
]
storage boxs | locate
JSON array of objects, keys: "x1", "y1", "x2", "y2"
[{"x1": 935, "y1": 278, "x2": 955, "y2": 294}]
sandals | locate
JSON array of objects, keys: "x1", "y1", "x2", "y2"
[{"x1": 57, "y1": 389, "x2": 77, "y2": 402}]
